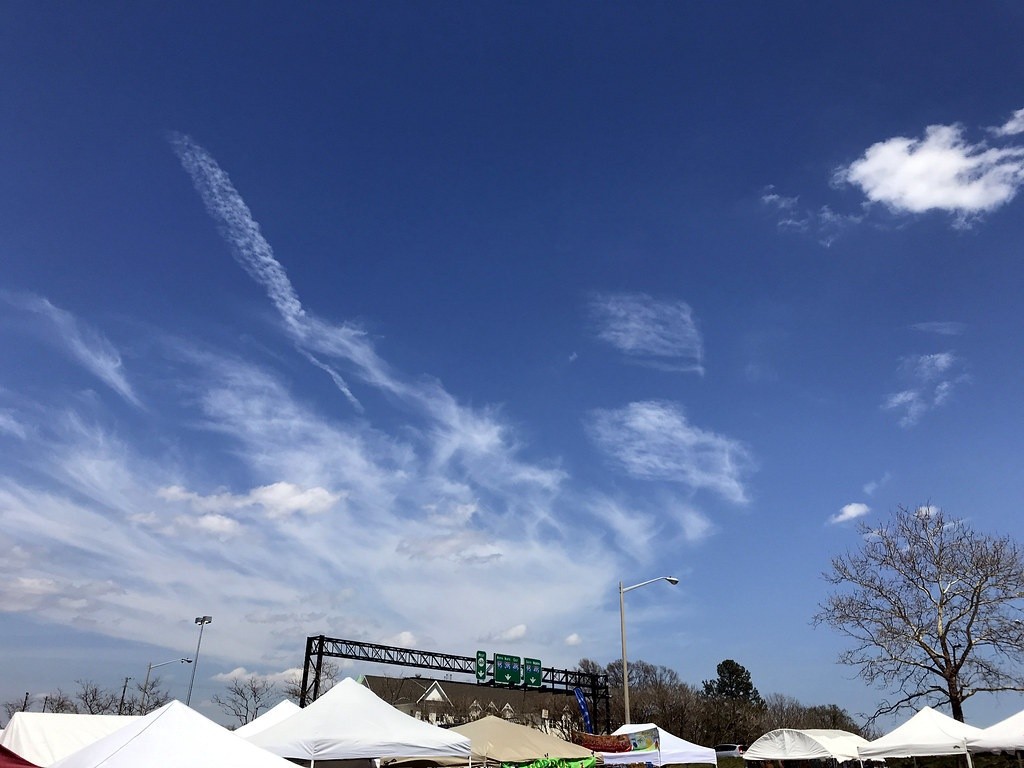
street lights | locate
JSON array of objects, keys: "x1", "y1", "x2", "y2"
[
  {"x1": 186, "y1": 615, "x2": 212, "y2": 706},
  {"x1": 619, "y1": 576, "x2": 679, "y2": 724},
  {"x1": 140, "y1": 658, "x2": 193, "y2": 716}
]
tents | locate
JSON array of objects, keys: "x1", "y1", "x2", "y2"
[
  {"x1": 0, "y1": 676, "x2": 718, "y2": 768},
  {"x1": 742, "y1": 729, "x2": 885, "y2": 768},
  {"x1": 963, "y1": 709, "x2": 1024, "y2": 768},
  {"x1": 857, "y1": 706, "x2": 995, "y2": 768}
]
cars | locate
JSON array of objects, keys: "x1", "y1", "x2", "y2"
[{"x1": 714, "y1": 744, "x2": 749, "y2": 758}]
